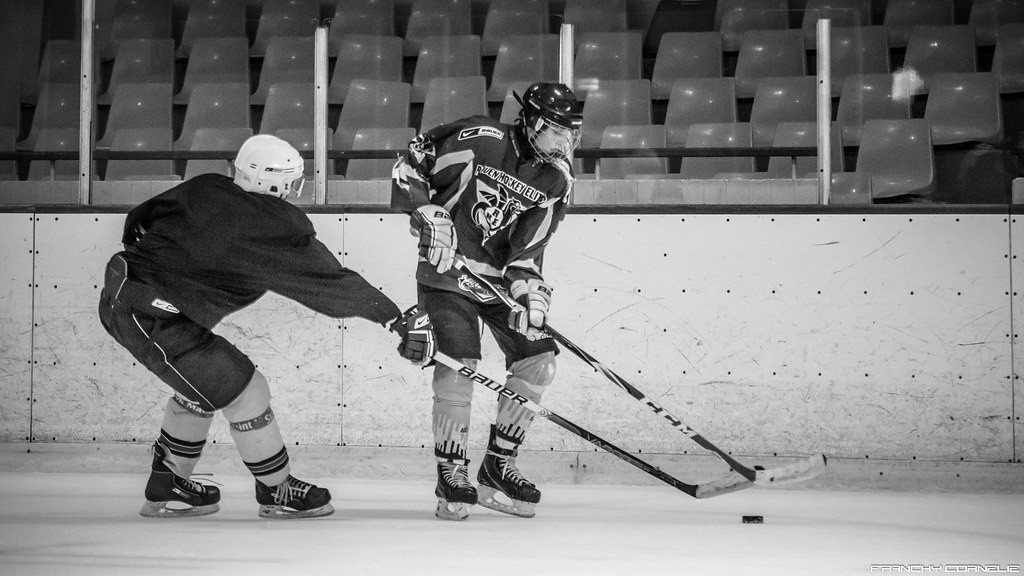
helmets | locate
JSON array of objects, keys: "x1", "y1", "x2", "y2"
[
  {"x1": 513, "y1": 82, "x2": 584, "y2": 142},
  {"x1": 234, "y1": 134, "x2": 305, "y2": 199}
]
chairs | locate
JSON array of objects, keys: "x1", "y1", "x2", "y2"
[
  {"x1": 836, "y1": 73, "x2": 911, "y2": 147},
  {"x1": 418, "y1": 76, "x2": 489, "y2": 135},
  {"x1": 333, "y1": 79, "x2": 410, "y2": 152},
  {"x1": 97, "y1": 83, "x2": 172, "y2": 150},
  {"x1": 680, "y1": 123, "x2": 757, "y2": 178},
  {"x1": 20, "y1": 0, "x2": 1024, "y2": 108},
  {"x1": 173, "y1": 83, "x2": 249, "y2": 149},
  {"x1": 596, "y1": 125, "x2": 668, "y2": 179},
  {"x1": 499, "y1": 81, "x2": 531, "y2": 125},
  {"x1": 768, "y1": 121, "x2": 934, "y2": 204},
  {"x1": 18, "y1": 85, "x2": 96, "y2": 150},
  {"x1": 580, "y1": 79, "x2": 651, "y2": 150},
  {"x1": 347, "y1": 128, "x2": 416, "y2": 180},
  {"x1": 184, "y1": 128, "x2": 254, "y2": 179},
  {"x1": 28, "y1": 128, "x2": 174, "y2": 181},
  {"x1": 750, "y1": 76, "x2": 817, "y2": 148},
  {"x1": 924, "y1": 72, "x2": 1004, "y2": 144},
  {"x1": 665, "y1": 77, "x2": 737, "y2": 149},
  {"x1": 275, "y1": 128, "x2": 333, "y2": 174},
  {"x1": 259, "y1": 83, "x2": 314, "y2": 136}
]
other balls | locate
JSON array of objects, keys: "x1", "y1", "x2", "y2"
[{"x1": 742, "y1": 515, "x2": 764, "y2": 524}]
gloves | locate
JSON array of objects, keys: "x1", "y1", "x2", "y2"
[
  {"x1": 410, "y1": 204, "x2": 458, "y2": 274},
  {"x1": 504, "y1": 266, "x2": 552, "y2": 341},
  {"x1": 393, "y1": 304, "x2": 438, "y2": 366}
]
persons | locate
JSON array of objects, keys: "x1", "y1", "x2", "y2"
[
  {"x1": 391, "y1": 81, "x2": 583, "y2": 504},
  {"x1": 98, "y1": 134, "x2": 437, "y2": 510}
]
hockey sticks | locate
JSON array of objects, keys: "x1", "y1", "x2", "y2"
[
  {"x1": 433, "y1": 352, "x2": 766, "y2": 499},
  {"x1": 419, "y1": 248, "x2": 828, "y2": 488}
]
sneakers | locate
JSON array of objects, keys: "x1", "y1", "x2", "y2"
[
  {"x1": 139, "y1": 441, "x2": 220, "y2": 518},
  {"x1": 477, "y1": 424, "x2": 541, "y2": 518},
  {"x1": 255, "y1": 474, "x2": 335, "y2": 519},
  {"x1": 435, "y1": 460, "x2": 478, "y2": 520}
]
